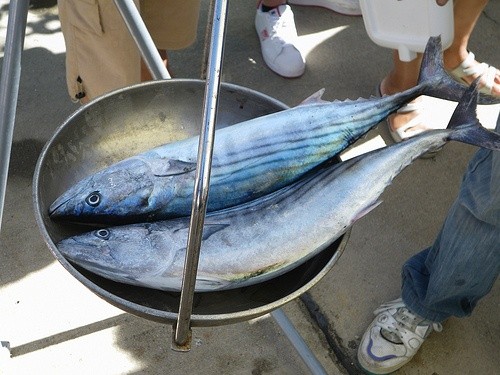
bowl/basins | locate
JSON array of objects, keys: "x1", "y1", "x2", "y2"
[{"x1": 32, "y1": 77, "x2": 352, "y2": 329}]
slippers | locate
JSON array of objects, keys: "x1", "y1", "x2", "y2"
[
  {"x1": 447, "y1": 49, "x2": 500, "y2": 100},
  {"x1": 375, "y1": 79, "x2": 437, "y2": 159}
]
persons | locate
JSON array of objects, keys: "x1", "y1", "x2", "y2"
[
  {"x1": 57, "y1": 0, "x2": 200, "y2": 108},
  {"x1": 255, "y1": 0, "x2": 363, "y2": 77},
  {"x1": 356, "y1": 109, "x2": 500, "y2": 375},
  {"x1": 376, "y1": 0, "x2": 500, "y2": 161}
]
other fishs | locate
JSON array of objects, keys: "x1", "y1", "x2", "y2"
[
  {"x1": 58, "y1": 73, "x2": 500, "y2": 294},
  {"x1": 48, "y1": 36, "x2": 500, "y2": 223}
]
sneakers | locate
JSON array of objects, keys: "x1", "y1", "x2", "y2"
[
  {"x1": 288, "y1": 0, "x2": 362, "y2": 16},
  {"x1": 255, "y1": 0, "x2": 305, "y2": 78},
  {"x1": 357, "y1": 296, "x2": 443, "y2": 375}
]
